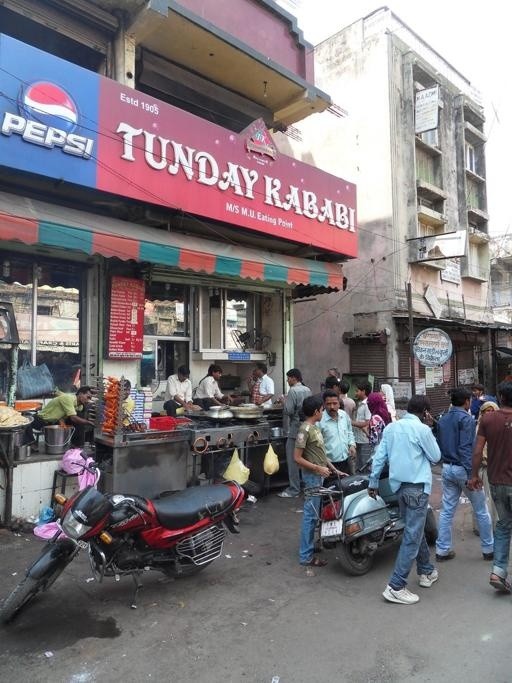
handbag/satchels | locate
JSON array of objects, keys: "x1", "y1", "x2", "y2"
[{"x1": 16, "y1": 363, "x2": 56, "y2": 399}]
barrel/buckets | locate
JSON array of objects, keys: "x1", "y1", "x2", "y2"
[{"x1": 40, "y1": 423, "x2": 76, "y2": 455}]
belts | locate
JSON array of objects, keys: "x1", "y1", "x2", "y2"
[{"x1": 443, "y1": 459, "x2": 461, "y2": 466}]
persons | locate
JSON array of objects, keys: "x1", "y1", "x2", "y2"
[
  {"x1": 247, "y1": 363, "x2": 275, "y2": 405},
  {"x1": 436, "y1": 387, "x2": 494, "y2": 561},
  {"x1": 368, "y1": 395, "x2": 442, "y2": 605},
  {"x1": 193, "y1": 364, "x2": 233, "y2": 410},
  {"x1": 471, "y1": 380, "x2": 512, "y2": 593},
  {"x1": 293, "y1": 396, "x2": 350, "y2": 566},
  {"x1": 119, "y1": 380, "x2": 134, "y2": 427},
  {"x1": 447, "y1": 385, "x2": 500, "y2": 537},
  {"x1": 26, "y1": 386, "x2": 96, "y2": 442},
  {"x1": 276, "y1": 368, "x2": 313, "y2": 498},
  {"x1": 163, "y1": 365, "x2": 193, "y2": 418},
  {"x1": 318, "y1": 367, "x2": 396, "y2": 487}
]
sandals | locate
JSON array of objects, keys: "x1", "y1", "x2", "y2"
[
  {"x1": 302, "y1": 557, "x2": 328, "y2": 566},
  {"x1": 489, "y1": 573, "x2": 512, "y2": 593}
]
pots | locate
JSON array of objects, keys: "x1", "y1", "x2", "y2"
[{"x1": 203, "y1": 409, "x2": 234, "y2": 423}]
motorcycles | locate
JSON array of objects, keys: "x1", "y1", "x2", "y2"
[
  {"x1": 301, "y1": 456, "x2": 443, "y2": 574},
  {"x1": 0, "y1": 440, "x2": 244, "y2": 627}
]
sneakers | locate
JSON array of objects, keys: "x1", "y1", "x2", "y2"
[
  {"x1": 383, "y1": 583, "x2": 420, "y2": 605},
  {"x1": 277, "y1": 492, "x2": 299, "y2": 498},
  {"x1": 419, "y1": 568, "x2": 439, "y2": 588},
  {"x1": 484, "y1": 551, "x2": 494, "y2": 560},
  {"x1": 436, "y1": 550, "x2": 455, "y2": 562}
]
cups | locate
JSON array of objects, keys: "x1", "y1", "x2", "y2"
[
  {"x1": 15, "y1": 446, "x2": 26, "y2": 461},
  {"x1": 272, "y1": 427, "x2": 280, "y2": 437},
  {"x1": 160, "y1": 410, "x2": 167, "y2": 417}
]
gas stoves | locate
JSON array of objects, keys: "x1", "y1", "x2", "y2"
[{"x1": 175, "y1": 419, "x2": 270, "y2": 448}]
